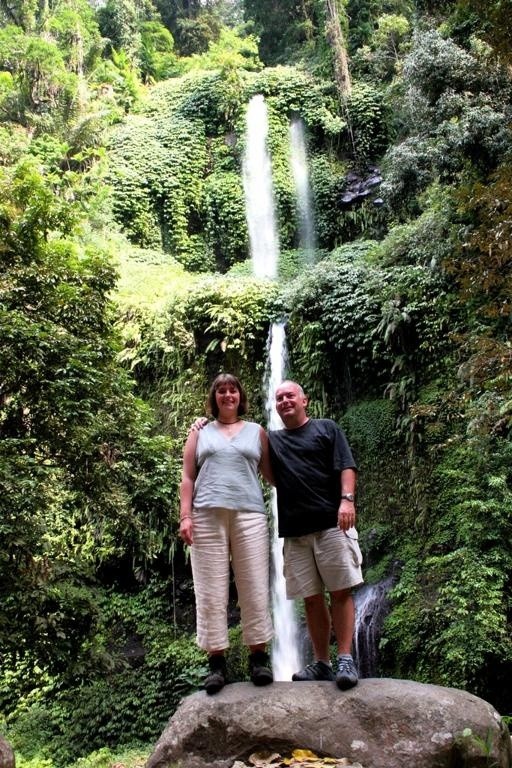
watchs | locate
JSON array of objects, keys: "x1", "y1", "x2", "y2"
[{"x1": 341, "y1": 493, "x2": 356, "y2": 501}]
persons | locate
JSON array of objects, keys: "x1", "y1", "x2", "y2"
[
  {"x1": 192, "y1": 381, "x2": 364, "y2": 686},
  {"x1": 180, "y1": 373, "x2": 275, "y2": 690}
]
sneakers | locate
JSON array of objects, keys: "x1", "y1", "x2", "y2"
[
  {"x1": 248, "y1": 655, "x2": 273, "y2": 682},
  {"x1": 204, "y1": 664, "x2": 228, "y2": 690},
  {"x1": 335, "y1": 657, "x2": 358, "y2": 690},
  {"x1": 292, "y1": 661, "x2": 335, "y2": 681}
]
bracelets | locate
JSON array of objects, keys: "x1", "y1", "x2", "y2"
[{"x1": 179, "y1": 516, "x2": 192, "y2": 523}]
